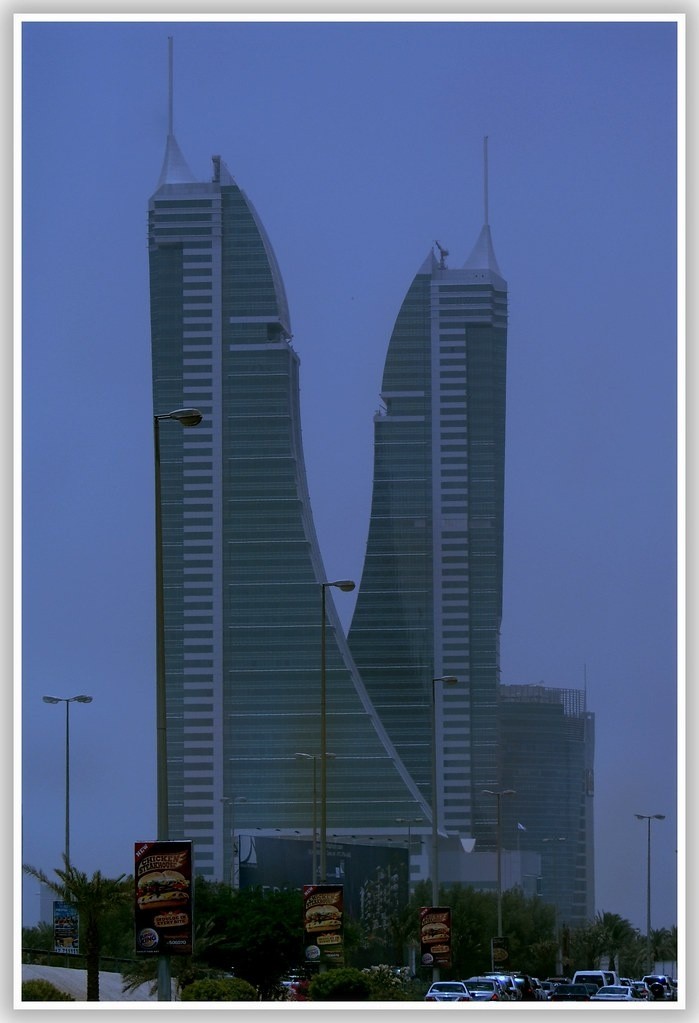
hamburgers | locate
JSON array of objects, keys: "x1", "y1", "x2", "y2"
[
  {"x1": 421, "y1": 923, "x2": 450, "y2": 944},
  {"x1": 305, "y1": 905, "x2": 343, "y2": 932},
  {"x1": 137, "y1": 870, "x2": 189, "y2": 910}
]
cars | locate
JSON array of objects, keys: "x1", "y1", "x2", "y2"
[
  {"x1": 547, "y1": 984, "x2": 590, "y2": 1001},
  {"x1": 607, "y1": 978, "x2": 633, "y2": 987},
  {"x1": 279, "y1": 968, "x2": 314, "y2": 990},
  {"x1": 423, "y1": 981, "x2": 475, "y2": 1001},
  {"x1": 387, "y1": 966, "x2": 422, "y2": 987},
  {"x1": 631, "y1": 981, "x2": 655, "y2": 1001},
  {"x1": 589, "y1": 985, "x2": 647, "y2": 1001},
  {"x1": 546, "y1": 978, "x2": 571, "y2": 986},
  {"x1": 462, "y1": 976, "x2": 554, "y2": 1002}
]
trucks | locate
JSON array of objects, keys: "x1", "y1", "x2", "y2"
[{"x1": 571, "y1": 970, "x2": 621, "y2": 994}]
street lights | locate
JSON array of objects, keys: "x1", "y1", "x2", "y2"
[
  {"x1": 432, "y1": 677, "x2": 458, "y2": 983},
  {"x1": 542, "y1": 837, "x2": 566, "y2": 977},
  {"x1": 295, "y1": 752, "x2": 337, "y2": 885},
  {"x1": 320, "y1": 579, "x2": 355, "y2": 886},
  {"x1": 397, "y1": 818, "x2": 422, "y2": 847},
  {"x1": 43, "y1": 694, "x2": 93, "y2": 900},
  {"x1": 482, "y1": 790, "x2": 515, "y2": 937},
  {"x1": 634, "y1": 814, "x2": 666, "y2": 976},
  {"x1": 221, "y1": 796, "x2": 247, "y2": 896},
  {"x1": 154, "y1": 408, "x2": 203, "y2": 1002}
]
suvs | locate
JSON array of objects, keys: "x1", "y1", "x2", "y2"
[{"x1": 642, "y1": 974, "x2": 677, "y2": 1001}]
